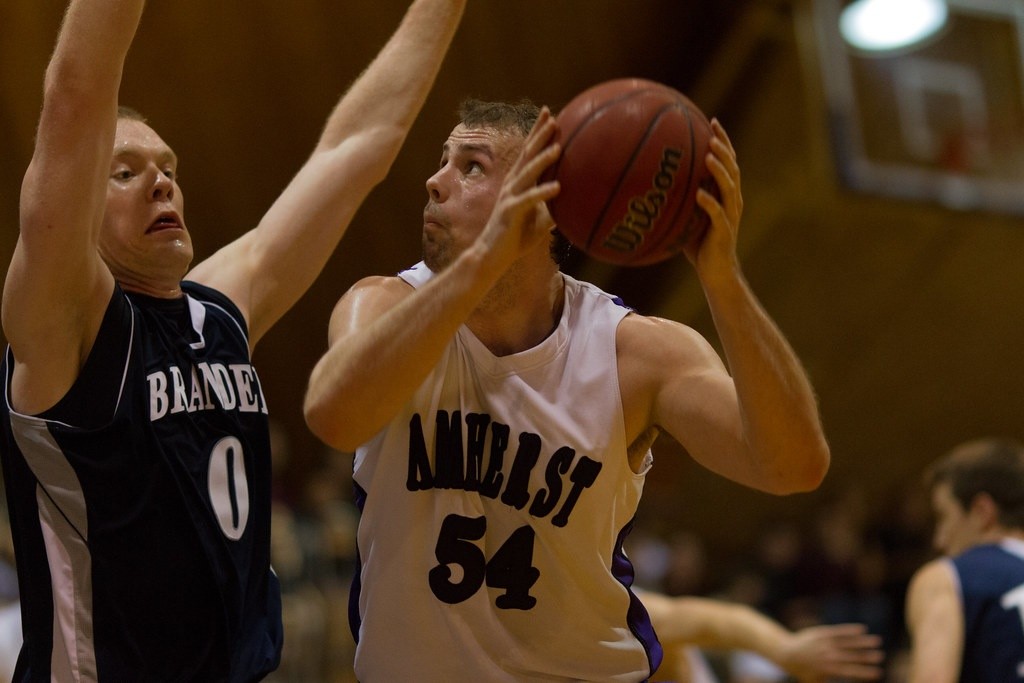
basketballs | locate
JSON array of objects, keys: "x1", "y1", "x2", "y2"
[{"x1": 531, "y1": 72, "x2": 723, "y2": 273}]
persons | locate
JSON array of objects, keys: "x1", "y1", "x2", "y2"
[
  {"x1": 303, "y1": 89, "x2": 1024, "y2": 682},
  {"x1": 0, "y1": 0, "x2": 466, "y2": 683}
]
